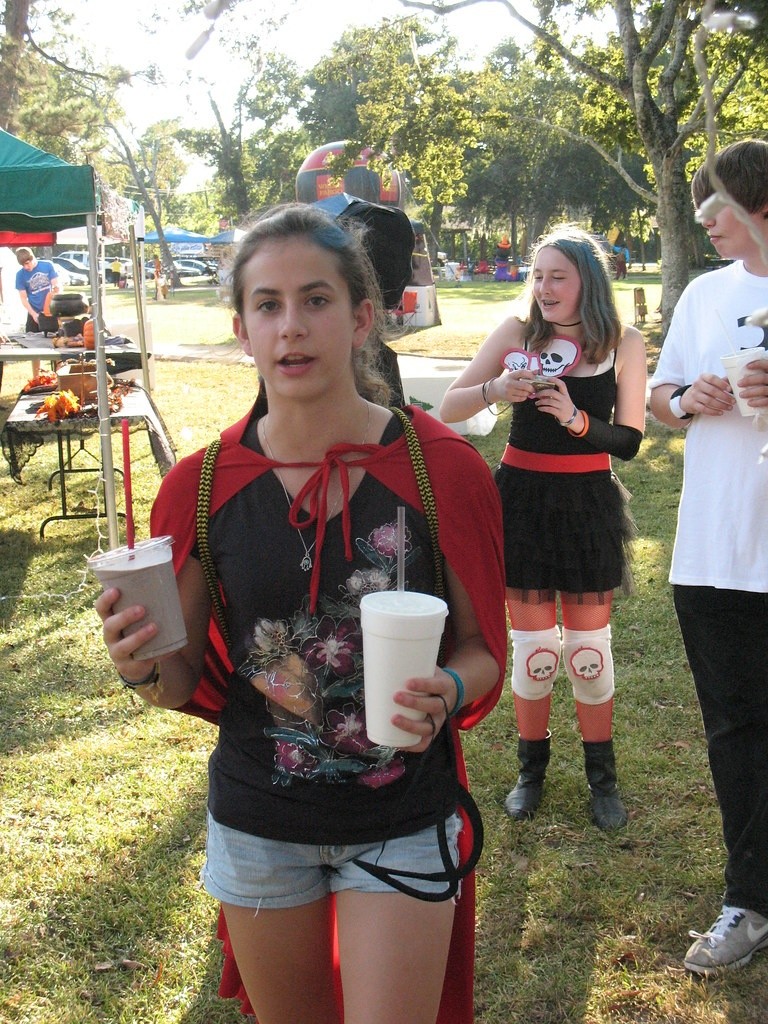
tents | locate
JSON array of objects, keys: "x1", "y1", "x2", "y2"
[
  {"x1": 0, "y1": 127, "x2": 151, "y2": 551},
  {"x1": 144, "y1": 226, "x2": 247, "y2": 244}
]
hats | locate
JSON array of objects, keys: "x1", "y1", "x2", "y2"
[{"x1": 15, "y1": 246, "x2": 34, "y2": 265}]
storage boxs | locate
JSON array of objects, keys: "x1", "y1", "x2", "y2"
[{"x1": 57, "y1": 364, "x2": 97, "y2": 402}]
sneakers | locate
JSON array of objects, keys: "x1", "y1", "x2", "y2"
[{"x1": 684, "y1": 905, "x2": 768, "y2": 974}]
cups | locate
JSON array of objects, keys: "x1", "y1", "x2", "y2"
[
  {"x1": 87, "y1": 535, "x2": 188, "y2": 661},
  {"x1": 359, "y1": 591, "x2": 449, "y2": 747},
  {"x1": 720, "y1": 347, "x2": 768, "y2": 417}
]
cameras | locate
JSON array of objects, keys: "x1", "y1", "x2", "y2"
[{"x1": 518, "y1": 375, "x2": 555, "y2": 394}]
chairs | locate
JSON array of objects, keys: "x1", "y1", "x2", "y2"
[
  {"x1": 393, "y1": 292, "x2": 418, "y2": 332},
  {"x1": 478, "y1": 261, "x2": 490, "y2": 281}
]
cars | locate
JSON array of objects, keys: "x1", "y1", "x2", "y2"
[{"x1": 52, "y1": 250, "x2": 221, "y2": 288}]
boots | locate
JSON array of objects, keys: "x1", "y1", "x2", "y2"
[
  {"x1": 580, "y1": 736, "x2": 628, "y2": 832},
  {"x1": 504, "y1": 729, "x2": 551, "y2": 824}
]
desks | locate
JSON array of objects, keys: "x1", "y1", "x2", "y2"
[
  {"x1": 0, "y1": 381, "x2": 177, "y2": 540},
  {"x1": 0, "y1": 340, "x2": 152, "y2": 391}
]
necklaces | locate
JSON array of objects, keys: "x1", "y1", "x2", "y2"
[
  {"x1": 553, "y1": 321, "x2": 581, "y2": 327},
  {"x1": 263, "y1": 399, "x2": 370, "y2": 571}
]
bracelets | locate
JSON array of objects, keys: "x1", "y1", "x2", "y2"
[
  {"x1": 443, "y1": 668, "x2": 464, "y2": 720},
  {"x1": 568, "y1": 410, "x2": 589, "y2": 437},
  {"x1": 555, "y1": 406, "x2": 578, "y2": 426},
  {"x1": 482, "y1": 377, "x2": 496, "y2": 405},
  {"x1": 670, "y1": 385, "x2": 693, "y2": 417},
  {"x1": 118, "y1": 660, "x2": 160, "y2": 691}
]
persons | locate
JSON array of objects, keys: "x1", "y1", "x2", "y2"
[
  {"x1": 611, "y1": 243, "x2": 629, "y2": 280},
  {"x1": 95, "y1": 205, "x2": 507, "y2": 1024},
  {"x1": 468, "y1": 259, "x2": 489, "y2": 273},
  {"x1": 15, "y1": 247, "x2": 61, "y2": 378},
  {"x1": 110, "y1": 258, "x2": 124, "y2": 287},
  {"x1": 650, "y1": 139, "x2": 768, "y2": 976},
  {"x1": 439, "y1": 228, "x2": 647, "y2": 832}
]
